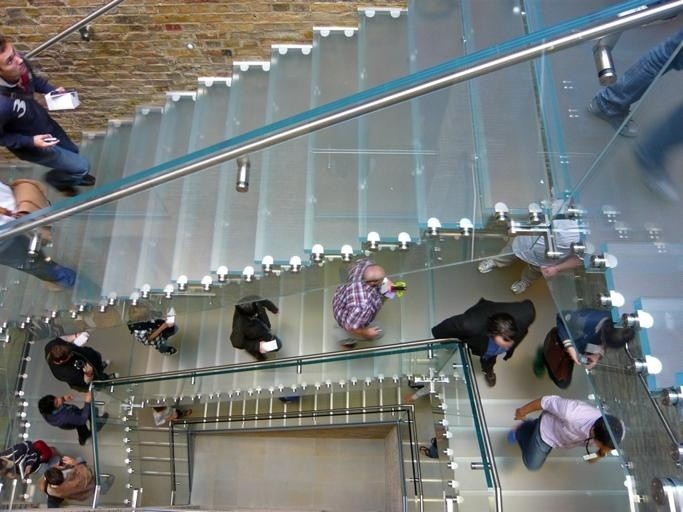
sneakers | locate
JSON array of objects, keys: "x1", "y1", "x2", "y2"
[
  {"x1": 511, "y1": 279, "x2": 531, "y2": 294},
  {"x1": 508, "y1": 418, "x2": 531, "y2": 444},
  {"x1": 184, "y1": 409, "x2": 193, "y2": 416},
  {"x1": 255, "y1": 353, "x2": 265, "y2": 361},
  {"x1": 478, "y1": 259, "x2": 496, "y2": 273},
  {"x1": 109, "y1": 373, "x2": 118, "y2": 379},
  {"x1": 272, "y1": 335, "x2": 281, "y2": 349},
  {"x1": 160, "y1": 346, "x2": 178, "y2": 355},
  {"x1": 341, "y1": 344, "x2": 355, "y2": 350},
  {"x1": 533, "y1": 347, "x2": 546, "y2": 378},
  {"x1": 42, "y1": 281, "x2": 66, "y2": 292},
  {"x1": 588, "y1": 100, "x2": 640, "y2": 138}
]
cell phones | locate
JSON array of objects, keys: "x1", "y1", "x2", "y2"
[{"x1": 51, "y1": 87, "x2": 76, "y2": 97}]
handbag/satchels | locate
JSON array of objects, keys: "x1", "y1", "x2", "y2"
[
  {"x1": 48, "y1": 495, "x2": 64, "y2": 508},
  {"x1": 12, "y1": 178, "x2": 50, "y2": 245},
  {"x1": 542, "y1": 328, "x2": 575, "y2": 389}
]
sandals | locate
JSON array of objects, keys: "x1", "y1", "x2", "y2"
[{"x1": 420, "y1": 446, "x2": 430, "y2": 456}]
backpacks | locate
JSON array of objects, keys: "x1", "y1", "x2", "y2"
[{"x1": 32, "y1": 440, "x2": 52, "y2": 463}]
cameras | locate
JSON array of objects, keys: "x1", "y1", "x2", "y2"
[{"x1": 579, "y1": 356, "x2": 589, "y2": 364}]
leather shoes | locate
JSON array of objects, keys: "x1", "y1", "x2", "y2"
[
  {"x1": 105, "y1": 475, "x2": 115, "y2": 487},
  {"x1": 42, "y1": 171, "x2": 96, "y2": 198},
  {"x1": 485, "y1": 371, "x2": 497, "y2": 389},
  {"x1": 80, "y1": 413, "x2": 108, "y2": 445}
]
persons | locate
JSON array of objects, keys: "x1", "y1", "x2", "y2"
[
  {"x1": 151, "y1": 407, "x2": 192, "y2": 426},
  {"x1": 278, "y1": 398, "x2": 301, "y2": 404},
  {"x1": 587, "y1": 27, "x2": 682, "y2": 204},
  {"x1": 125, "y1": 299, "x2": 181, "y2": 356},
  {"x1": 403, "y1": 382, "x2": 437, "y2": 405},
  {"x1": 420, "y1": 431, "x2": 448, "y2": 459},
  {"x1": 508, "y1": 395, "x2": 625, "y2": 472},
  {"x1": 0, "y1": 36, "x2": 95, "y2": 197},
  {"x1": 432, "y1": 219, "x2": 633, "y2": 390},
  {"x1": 0, "y1": 332, "x2": 119, "y2": 501},
  {"x1": 230, "y1": 296, "x2": 282, "y2": 361},
  {"x1": 333, "y1": 257, "x2": 394, "y2": 351},
  {"x1": 0, "y1": 177, "x2": 75, "y2": 295}
]
